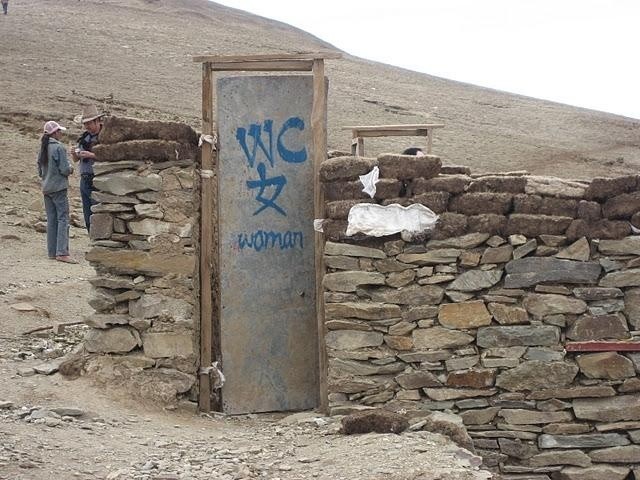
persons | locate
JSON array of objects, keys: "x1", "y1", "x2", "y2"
[
  {"x1": 0, "y1": 0, "x2": 10, "y2": 15},
  {"x1": 402, "y1": 148, "x2": 425, "y2": 156},
  {"x1": 71, "y1": 103, "x2": 103, "y2": 234},
  {"x1": 37, "y1": 121, "x2": 78, "y2": 263}
]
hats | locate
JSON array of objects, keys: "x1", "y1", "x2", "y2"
[
  {"x1": 73, "y1": 105, "x2": 104, "y2": 123},
  {"x1": 44, "y1": 121, "x2": 66, "y2": 134}
]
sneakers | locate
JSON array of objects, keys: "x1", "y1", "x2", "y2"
[{"x1": 56, "y1": 256, "x2": 76, "y2": 264}]
